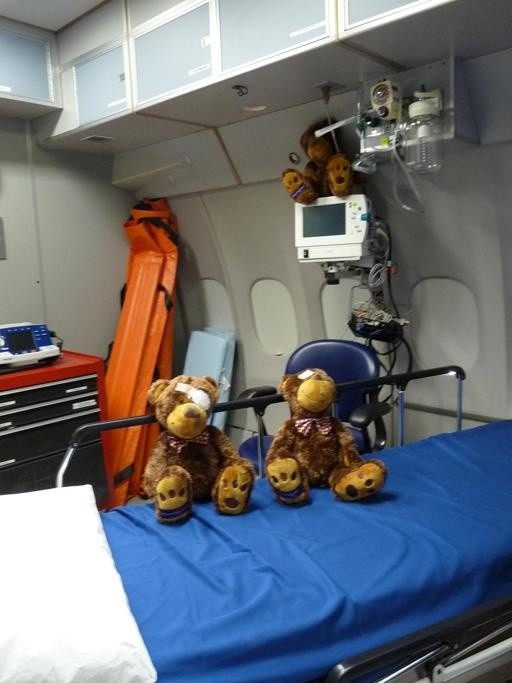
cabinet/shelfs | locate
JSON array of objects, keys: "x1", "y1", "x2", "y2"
[{"x1": 0, "y1": 351, "x2": 117, "y2": 510}]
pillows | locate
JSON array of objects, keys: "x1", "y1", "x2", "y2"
[{"x1": 1, "y1": 482, "x2": 158, "y2": 683}]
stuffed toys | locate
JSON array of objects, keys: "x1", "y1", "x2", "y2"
[
  {"x1": 265, "y1": 366, "x2": 390, "y2": 505},
  {"x1": 143, "y1": 374, "x2": 255, "y2": 525},
  {"x1": 280, "y1": 115, "x2": 353, "y2": 203}
]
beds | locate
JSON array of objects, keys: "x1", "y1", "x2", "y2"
[{"x1": 1, "y1": 365, "x2": 511, "y2": 682}]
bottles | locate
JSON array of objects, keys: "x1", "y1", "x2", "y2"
[{"x1": 403, "y1": 102, "x2": 444, "y2": 172}]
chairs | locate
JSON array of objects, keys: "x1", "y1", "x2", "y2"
[{"x1": 236, "y1": 340, "x2": 393, "y2": 479}]
